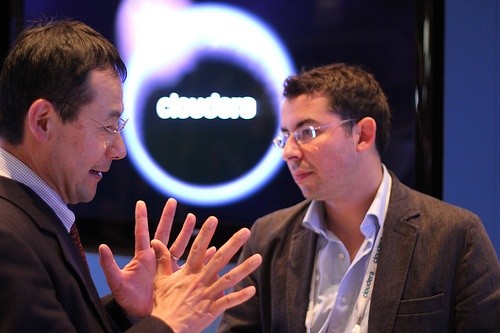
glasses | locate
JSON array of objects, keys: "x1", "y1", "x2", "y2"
[
  {"x1": 58, "y1": 100, "x2": 130, "y2": 143},
  {"x1": 272, "y1": 117, "x2": 359, "y2": 147}
]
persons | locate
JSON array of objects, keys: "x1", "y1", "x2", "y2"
[
  {"x1": 1, "y1": 14, "x2": 263, "y2": 333},
  {"x1": 219, "y1": 59, "x2": 500, "y2": 333}
]
